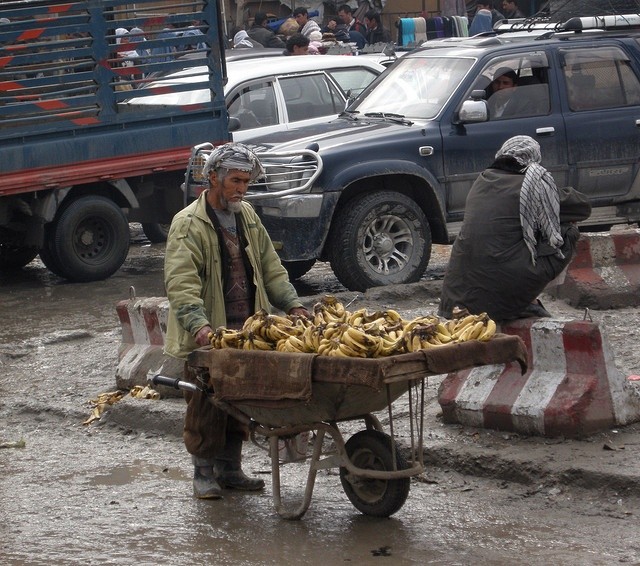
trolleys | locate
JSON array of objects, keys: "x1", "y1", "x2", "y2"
[{"x1": 153, "y1": 314, "x2": 528, "y2": 520}]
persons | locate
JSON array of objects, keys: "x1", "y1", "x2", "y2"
[
  {"x1": 230, "y1": 25, "x2": 264, "y2": 48},
  {"x1": 115, "y1": 28, "x2": 139, "y2": 68},
  {"x1": 500, "y1": 0, "x2": 522, "y2": 17},
  {"x1": 128, "y1": 27, "x2": 151, "y2": 62},
  {"x1": 281, "y1": 34, "x2": 311, "y2": 55},
  {"x1": 486, "y1": 67, "x2": 530, "y2": 114},
  {"x1": 469, "y1": 10, "x2": 494, "y2": 36},
  {"x1": 293, "y1": 7, "x2": 321, "y2": 40},
  {"x1": 246, "y1": 12, "x2": 286, "y2": 48},
  {"x1": 178, "y1": 26, "x2": 208, "y2": 49},
  {"x1": 151, "y1": 29, "x2": 176, "y2": 62},
  {"x1": 321, "y1": 19, "x2": 337, "y2": 34},
  {"x1": 162, "y1": 141, "x2": 312, "y2": 500},
  {"x1": 477, "y1": 1, "x2": 505, "y2": 26},
  {"x1": 438, "y1": 135, "x2": 593, "y2": 319},
  {"x1": 227, "y1": 92, "x2": 262, "y2": 130},
  {"x1": 338, "y1": 5, "x2": 366, "y2": 34},
  {"x1": 363, "y1": 9, "x2": 391, "y2": 43}
]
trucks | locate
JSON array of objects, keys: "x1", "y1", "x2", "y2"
[{"x1": 0, "y1": 0, "x2": 229, "y2": 281}]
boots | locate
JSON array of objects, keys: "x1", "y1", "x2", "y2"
[
  {"x1": 192, "y1": 456, "x2": 222, "y2": 498},
  {"x1": 218, "y1": 454, "x2": 265, "y2": 491}
]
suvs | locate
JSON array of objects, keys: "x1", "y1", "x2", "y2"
[{"x1": 180, "y1": 6, "x2": 640, "y2": 293}]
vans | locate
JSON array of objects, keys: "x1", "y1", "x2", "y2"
[
  {"x1": 122, "y1": 55, "x2": 388, "y2": 243},
  {"x1": 125, "y1": 45, "x2": 322, "y2": 109}
]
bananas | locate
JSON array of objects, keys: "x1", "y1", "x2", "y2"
[{"x1": 206, "y1": 294, "x2": 498, "y2": 363}]
